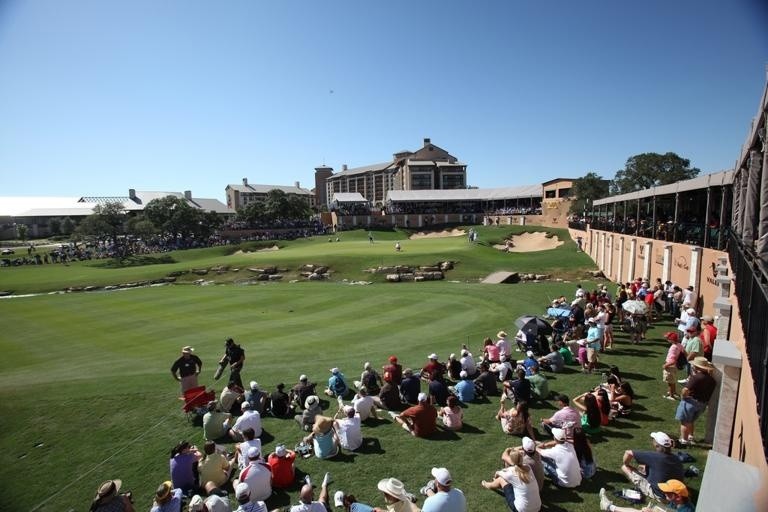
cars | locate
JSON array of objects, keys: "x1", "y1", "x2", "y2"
[
  {"x1": 86, "y1": 233, "x2": 182, "y2": 246},
  {"x1": 1, "y1": 249, "x2": 15, "y2": 255},
  {"x1": 230, "y1": 220, "x2": 321, "y2": 229}
]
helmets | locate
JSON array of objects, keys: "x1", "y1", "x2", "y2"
[{"x1": 521, "y1": 435, "x2": 537, "y2": 453}]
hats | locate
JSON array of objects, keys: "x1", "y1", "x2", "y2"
[
  {"x1": 430, "y1": 466, "x2": 452, "y2": 487},
  {"x1": 207, "y1": 351, "x2": 471, "y2": 434},
  {"x1": 657, "y1": 479, "x2": 689, "y2": 498},
  {"x1": 483, "y1": 277, "x2": 720, "y2": 446},
  {"x1": 333, "y1": 490, "x2": 345, "y2": 507},
  {"x1": 92, "y1": 441, "x2": 316, "y2": 510},
  {"x1": 376, "y1": 476, "x2": 410, "y2": 503},
  {"x1": 650, "y1": 431, "x2": 673, "y2": 448},
  {"x1": 550, "y1": 427, "x2": 567, "y2": 442},
  {"x1": 181, "y1": 345, "x2": 193, "y2": 353}
]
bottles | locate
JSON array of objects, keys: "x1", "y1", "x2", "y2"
[{"x1": 298, "y1": 442, "x2": 311, "y2": 459}]
